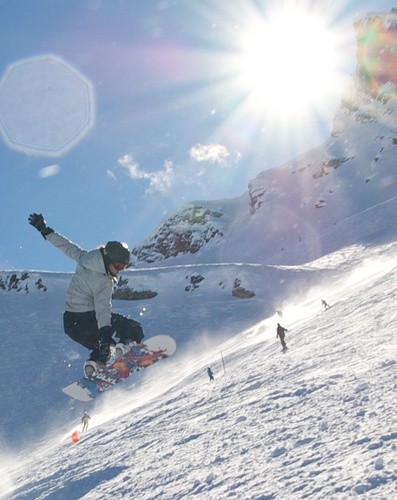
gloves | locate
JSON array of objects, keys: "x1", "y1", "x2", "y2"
[
  {"x1": 27, "y1": 212, "x2": 54, "y2": 240},
  {"x1": 93, "y1": 326, "x2": 110, "y2": 356}
]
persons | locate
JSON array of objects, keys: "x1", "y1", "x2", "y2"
[
  {"x1": 275, "y1": 323, "x2": 288, "y2": 353},
  {"x1": 80, "y1": 411, "x2": 90, "y2": 431},
  {"x1": 27, "y1": 212, "x2": 145, "y2": 378},
  {"x1": 206, "y1": 367, "x2": 215, "y2": 382},
  {"x1": 321, "y1": 299, "x2": 329, "y2": 310}
]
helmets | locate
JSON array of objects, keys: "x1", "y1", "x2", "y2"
[{"x1": 104, "y1": 241, "x2": 129, "y2": 264}]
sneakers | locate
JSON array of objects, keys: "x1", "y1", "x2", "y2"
[
  {"x1": 114, "y1": 342, "x2": 131, "y2": 356},
  {"x1": 82, "y1": 360, "x2": 100, "y2": 379}
]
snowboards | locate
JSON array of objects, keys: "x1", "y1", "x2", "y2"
[{"x1": 61, "y1": 334, "x2": 177, "y2": 402}]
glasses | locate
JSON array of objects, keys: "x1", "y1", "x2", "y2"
[{"x1": 111, "y1": 261, "x2": 126, "y2": 271}]
